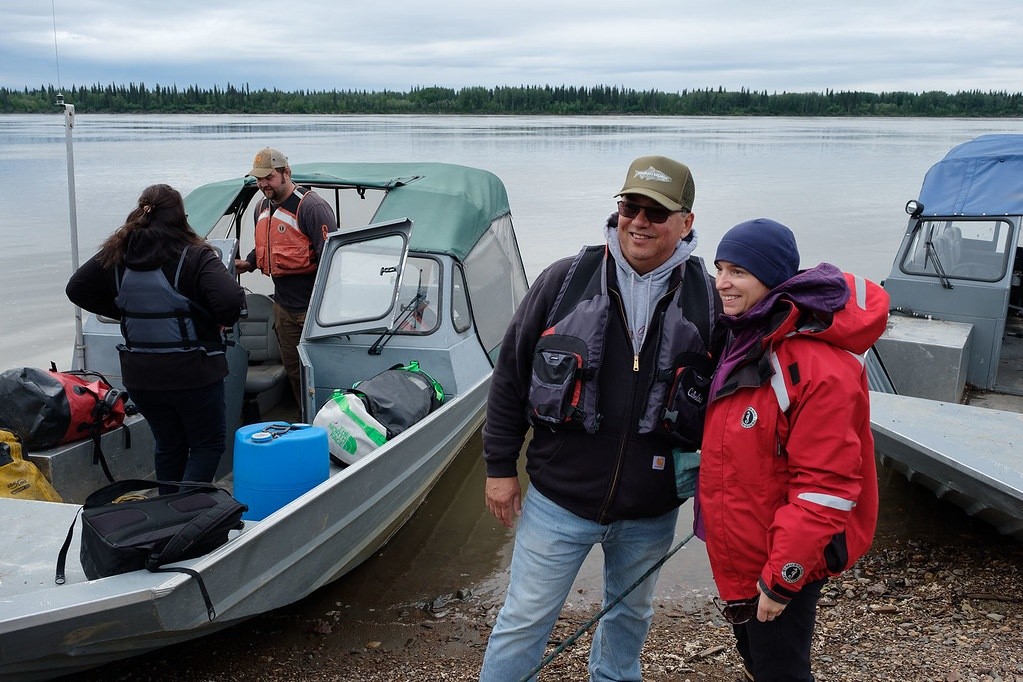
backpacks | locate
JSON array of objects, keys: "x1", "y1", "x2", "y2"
[
  {"x1": 0, "y1": 428, "x2": 65, "y2": 504},
  {"x1": 0, "y1": 361, "x2": 133, "y2": 484},
  {"x1": 53, "y1": 479, "x2": 249, "y2": 624},
  {"x1": 311, "y1": 359, "x2": 446, "y2": 469}
]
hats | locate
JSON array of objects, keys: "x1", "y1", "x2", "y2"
[
  {"x1": 713, "y1": 217, "x2": 800, "y2": 290},
  {"x1": 613, "y1": 155, "x2": 696, "y2": 212},
  {"x1": 244, "y1": 146, "x2": 288, "y2": 178}
]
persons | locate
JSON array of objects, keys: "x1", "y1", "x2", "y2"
[
  {"x1": 234, "y1": 146, "x2": 340, "y2": 415},
  {"x1": 474, "y1": 155, "x2": 723, "y2": 682},
  {"x1": 695, "y1": 217, "x2": 890, "y2": 681},
  {"x1": 64, "y1": 183, "x2": 240, "y2": 502}
]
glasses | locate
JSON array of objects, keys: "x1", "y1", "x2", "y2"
[
  {"x1": 617, "y1": 200, "x2": 690, "y2": 224},
  {"x1": 713, "y1": 594, "x2": 761, "y2": 626}
]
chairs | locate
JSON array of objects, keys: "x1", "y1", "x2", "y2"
[
  {"x1": 232, "y1": 293, "x2": 288, "y2": 391},
  {"x1": 914, "y1": 227, "x2": 1002, "y2": 280}
]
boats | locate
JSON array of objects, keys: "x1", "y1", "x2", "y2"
[
  {"x1": 842, "y1": 130, "x2": 1023, "y2": 537},
  {"x1": 0, "y1": 162, "x2": 539, "y2": 682}
]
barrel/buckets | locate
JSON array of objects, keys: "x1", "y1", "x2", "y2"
[{"x1": 234, "y1": 422, "x2": 330, "y2": 520}]
